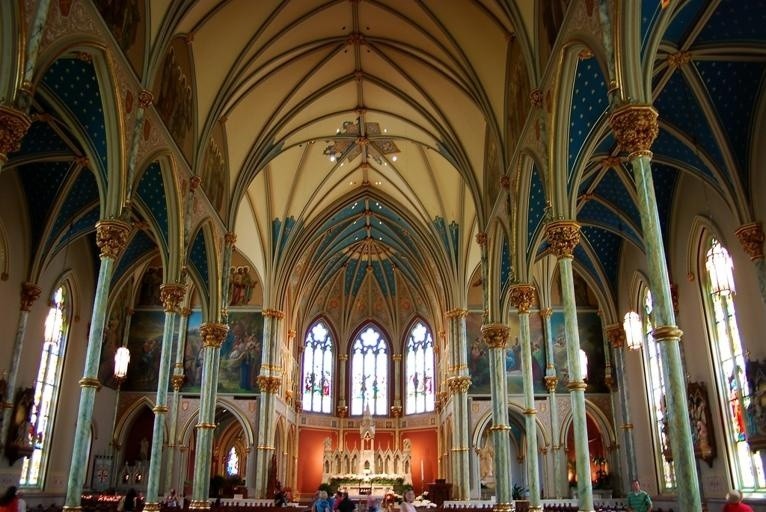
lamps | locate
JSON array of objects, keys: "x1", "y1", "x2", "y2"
[
  {"x1": 580, "y1": 349, "x2": 588, "y2": 379},
  {"x1": 113, "y1": 289, "x2": 131, "y2": 377},
  {"x1": 613, "y1": 185, "x2": 644, "y2": 350},
  {"x1": 45, "y1": 215, "x2": 74, "y2": 347}
]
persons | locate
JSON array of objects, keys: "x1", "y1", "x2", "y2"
[
  {"x1": 722, "y1": 488, "x2": 755, "y2": 511},
  {"x1": 117, "y1": 487, "x2": 144, "y2": 512},
  {"x1": 624, "y1": 479, "x2": 653, "y2": 511},
  {"x1": 0, "y1": 485, "x2": 27, "y2": 512},
  {"x1": 129, "y1": 331, "x2": 261, "y2": 392},
  {"x1": 470, "y1": 333, "x2": 547, "y2": 383},
  {"x1": 162, "y1": 488, "x2": 180, "y2": 508},
  {"x1": 310, "y1": 488, "x2": 417, "y2": 512}
]
